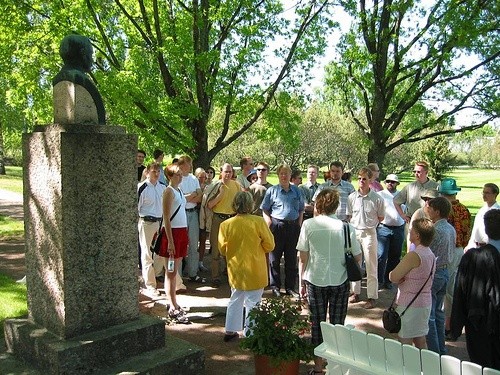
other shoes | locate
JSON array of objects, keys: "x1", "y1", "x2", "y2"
[
  {"x1": 348, "y1": 293, "x2": 360, "y2": 302},
  {"x1": 378, "y1": 282, "x2": 392, "y2": 289},
  {"x1": 272, "y1": 289, "x2": 281, "y2": 298},
  {"x1": 445, "y1": 327, "x2": 462, "y2": 342},
  {"x1": 157, "y1": 264, "x2": 228, "y2": 294},
  {"x1": 169, "y1": 305, "x2": 189, "y2": 324},
  {"x1": 286, "y1": 288, "x2": 300, "y2": 299},
  {"x1": 224, "y1": 332, "x2": 239, "y2": 341},
  {"x1": 364, "y1": 298, "x2": 376, "y2": 309},
  {"x1": 147, "y1": 288, "x2": 161, "y2": 296}
]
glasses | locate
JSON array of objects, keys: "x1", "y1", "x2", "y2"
[
  {"x1": 252, "y1": 177, "x2": 258, "y2": 180},
  {"x1": 257, "y1": 168, "x2": 266, "y2": 172},
  {"x1": 358, "y1": 171, "x2": 421, "y2": 183},
  {"x1": 424, "y1": 198, "x2": 433, "y2": 202},
  {"x1": 207, "y1": 171, "x2": 213, "y2": 174}
]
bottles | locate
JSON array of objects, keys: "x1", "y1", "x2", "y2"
[{"x1": 167, "y1": 253, "x2": 175, "y2": 272}]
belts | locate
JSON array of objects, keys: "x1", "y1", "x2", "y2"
[
  {"x1": 216, "y1": 213, "x2": 237, "y2": 218},
  {"x1": 185, "y1": 206, "x2": 198, "y2": 212},
  {"x1": 141, "y1": 217, "x2": 162, "y2": 221}
]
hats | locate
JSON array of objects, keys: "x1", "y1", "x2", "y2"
[
  {"x1": 421, "y1": 190, "x2": 440, "y2": 201},
  {"x1": 384, "y1": 174, "x2": 400, "y2": 185},
  {"x1": 438, "y1": 179, "x2": 461, "y2": 195},
  {"x1": 247, "y1": 169, "x2": 257, "y2": 183},
  {"x1": 153, "y1": 150, "x2": 163, "y2": 159}
]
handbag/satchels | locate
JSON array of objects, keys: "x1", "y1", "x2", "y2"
[
  {"x1": 344, "y1": 223, "x2": 363, "y2": 281},
  {"x1": 383, "y1": 307, "x2": 402, "y2": 334},
  {"x1": 149, "y1": 231, "x2": 163, "y2": 255}
]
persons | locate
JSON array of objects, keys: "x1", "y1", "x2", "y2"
[
  {"x1": 52, "y1": 34, "x2": 106, "y2": 124},
  {"x1": 296, "y1": 186, "x2": 363, "y2": 375},
  {"x1": 382, "y1": 218, "x2": 436, "y2": 351},
  {"x1": 134, "y1": 150, "x2": 500, "y2": 370},
  {"x1": 218, "y1": 190, "x2": 276, "y2": 342}
]
